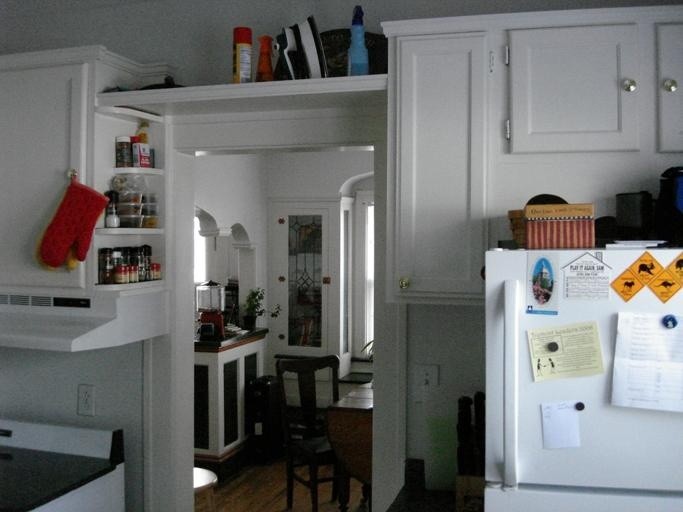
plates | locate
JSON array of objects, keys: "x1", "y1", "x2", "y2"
[{"x1": 612, "y1": 236, "x2": 668, "y2": 248}]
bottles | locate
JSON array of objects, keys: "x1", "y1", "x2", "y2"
[
  {"x1": 233, "y1": 26, "x2": 253, "y2": 83},
  {"x1": 104, "y1": 190, "x2": 121, "y2": 228},
  {"x1": 97, "y1": 244, "x2": 160, "y2": 284},
  {"x1": 116, "y1": 122, "x2": 152, "y2": 167}
]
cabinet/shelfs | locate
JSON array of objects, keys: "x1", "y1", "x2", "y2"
[
  {"x1": 381, "y1": 13, "x2": 494, "y2": 304},
  {"x1": 0, "y1": 45, "x2": 172, "y2": 352},
  {"x1": 501, "y1": 4, "x2": 681, "y2": 158}
]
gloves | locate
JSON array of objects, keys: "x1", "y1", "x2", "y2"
[{"x1": 32, "y1": 179, "x2": 110, "y2": 274}]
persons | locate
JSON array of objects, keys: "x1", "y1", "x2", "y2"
[{"x1": 293, "y1": 287, "x2": 320, "y2": 346}]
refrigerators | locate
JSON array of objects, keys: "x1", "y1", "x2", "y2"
[{"x1": 480, "y1": 249, "x2": 683, "y2": 510}]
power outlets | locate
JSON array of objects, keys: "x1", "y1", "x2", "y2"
[{"x1": 78, "y1": 384, "x2": 97, "y2": 417}]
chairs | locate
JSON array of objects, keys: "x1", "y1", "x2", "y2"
[{"x1": 274, "y1": 354, "x2": 340, "y2": 512}]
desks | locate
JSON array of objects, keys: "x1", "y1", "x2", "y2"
[{"x1": 327, "y1": 382, "x2": 372, "y2": 511}]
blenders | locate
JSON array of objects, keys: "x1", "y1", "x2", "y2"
[{"x1": 196, "y1": 280, "x2": 226, "y2": 341}]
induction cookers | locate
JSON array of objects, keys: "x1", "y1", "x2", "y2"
[{"x1": 0, "y1": 444, "x2": 111, "y2": 512}]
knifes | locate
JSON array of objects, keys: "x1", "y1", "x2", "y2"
[{"x1": 456, "y1": 390, "x2": 486, "y2": 478}]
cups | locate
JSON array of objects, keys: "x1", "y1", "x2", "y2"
[
  {"x1": 199, "y1": 323, "x2": 215, "y2": 341},
  {"x1": 614, "y1": 190, "x2": 654, "y2": 238}
]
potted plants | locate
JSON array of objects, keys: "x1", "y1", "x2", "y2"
[{"x1": 241, "y1": 287, "x2": 279, "y2": 330}]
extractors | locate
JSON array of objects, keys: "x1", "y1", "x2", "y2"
[{"x1": 0, "y1": 285, "x2": 169, "y2": 354}]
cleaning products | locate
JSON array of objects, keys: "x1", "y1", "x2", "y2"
[{"x1": 348, "y1": 3, "x2": 371, "y2": 75}]
